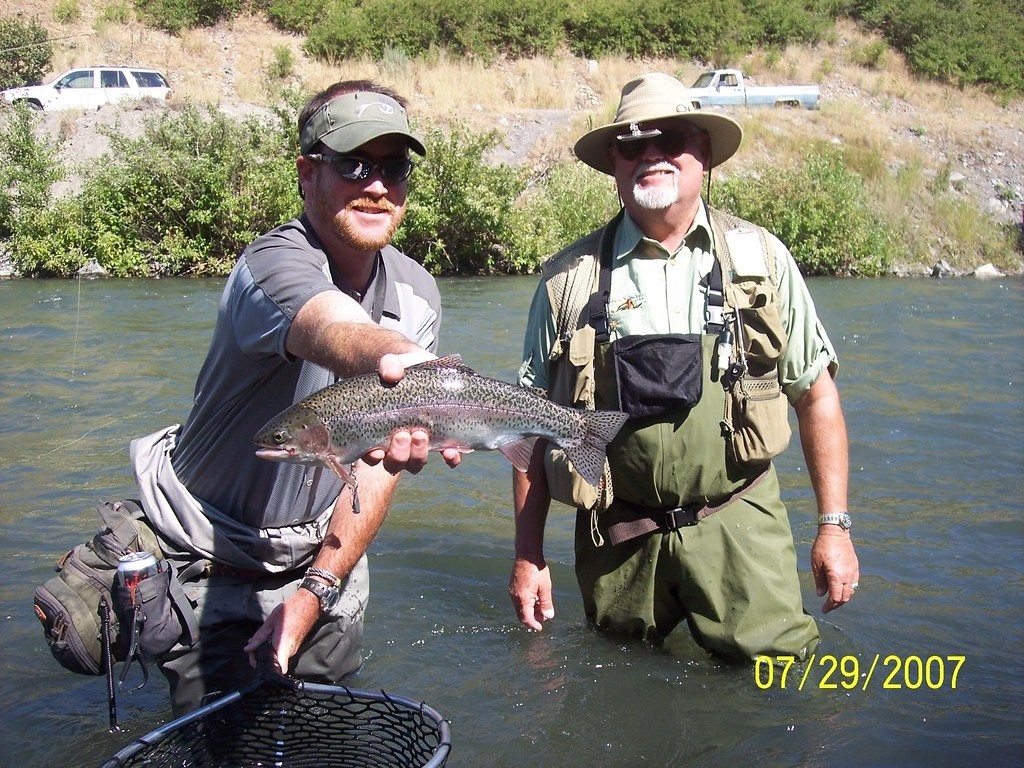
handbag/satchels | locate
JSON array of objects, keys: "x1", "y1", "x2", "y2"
[
  {"x1": 34, "y1": 498, "x2": 230, "y2": 676},
  {"x1": 613, "y1": 333, "x2": 703, "y2": 421}
]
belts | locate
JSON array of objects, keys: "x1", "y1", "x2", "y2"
[{"x1": 599, "y1": 460, "x2": 771, "y2": 547}]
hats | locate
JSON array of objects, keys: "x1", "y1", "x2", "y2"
[
  {"x1": 574, "y1": 73, "x2": 743, "y2": 177},
  {"x1": 300, "y1": 92, "x2": 427, "y2": 156}
]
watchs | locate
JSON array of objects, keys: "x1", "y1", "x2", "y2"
[
  {"x1": 817, "y1": 511, "x2": 852, "y2": 530},
  {"x1": 296, "y1": 576, "x2": 341, "y2": 615}
]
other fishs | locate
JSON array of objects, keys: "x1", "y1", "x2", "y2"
[{"x1": 252, "y1": 353, "x2": 629, "y2": 488}]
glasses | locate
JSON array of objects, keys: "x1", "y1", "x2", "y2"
[
  {"x1": 618, "y1": 129, "x2": 699, "y2": 160},
  {"x1": 305, "y1": 153, "x2": 415, "y2": 186}
]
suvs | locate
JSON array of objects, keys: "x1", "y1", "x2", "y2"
[{"x1": 0, "y1": 64, "x2": 175, "y2": 112}]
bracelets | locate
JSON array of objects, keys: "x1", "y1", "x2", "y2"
[{"x1": 304, "y1": 567, "x2": 342, "y2": 590}]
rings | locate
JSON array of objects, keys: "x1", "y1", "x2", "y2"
[{"x1": 851, "y1": 582, "x2": 859, "y2": 591}]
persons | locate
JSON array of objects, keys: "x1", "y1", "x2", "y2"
[
  {"x1": 126, "y1": 79, "x2": 461, "y2": 766},
  {"x1": 509, "y1": 73, "x2": 861, "y2": 674}
]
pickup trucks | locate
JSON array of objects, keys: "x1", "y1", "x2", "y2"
[{"x1": 687, "y1": 69, "x2": 821, "y2": 111}]
